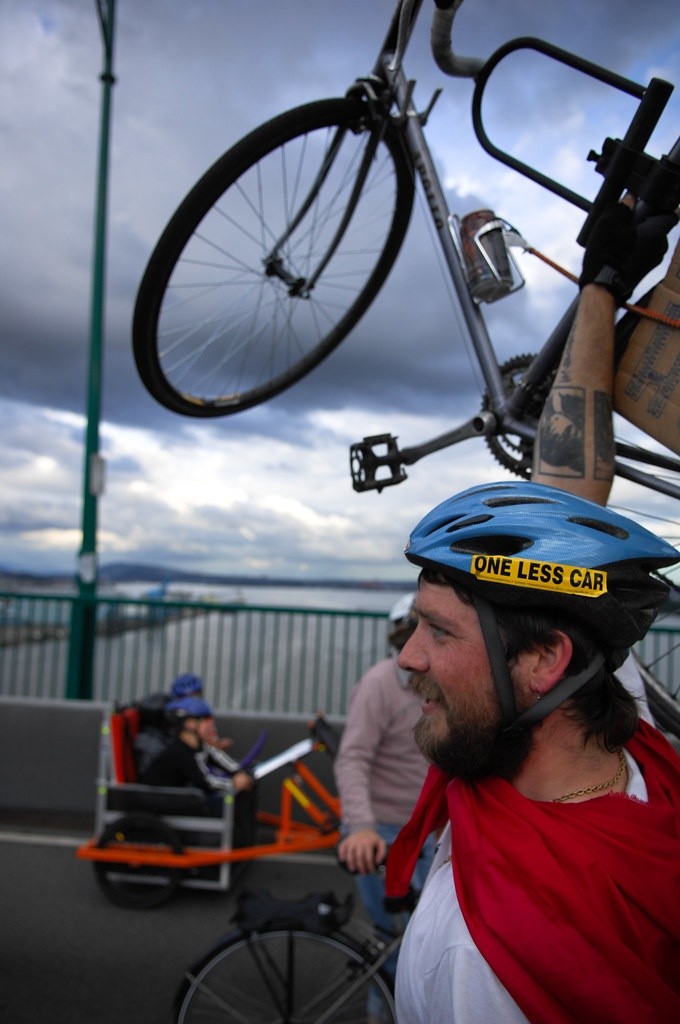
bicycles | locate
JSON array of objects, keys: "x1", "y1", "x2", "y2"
[
  {"x1": 172, "y1": 843, "x2": 427, "y2": 1024},
  {"x1": 129, "y1": 1, "x2": 680, "y2": 742}
]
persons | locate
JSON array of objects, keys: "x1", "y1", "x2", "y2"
[
  {"x1": 395, "y1": 189, "x2": 680, "y2": 1024},
  {"x1": 149, "y1": 592, "x2": 448, "y2": 1024}
]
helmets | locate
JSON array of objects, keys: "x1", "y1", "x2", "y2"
[
  {"x1": 404, "y1": 481, "x2": 680, "y2": 615},
  {"x1": 164, "y1": 697, "x2": 213, "y2": 718},
  {"x1": 391, "y1": 590, "x2": 418, "y2": 621},
  {"x1": 173, "y1": 674, "x2": 204, "y2": 695}
]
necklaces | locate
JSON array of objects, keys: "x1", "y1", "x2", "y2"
[{"x1": 549, "y1": 746, "x2": 628, "y2": 802}]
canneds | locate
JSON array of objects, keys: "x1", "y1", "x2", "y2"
[{"x1": 460, "y1": 209, "x2": 513, "y2": 300}]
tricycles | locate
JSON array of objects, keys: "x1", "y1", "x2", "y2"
[{"x1": 75, "y1": 689, "x2": 342, "y2": 909}]
